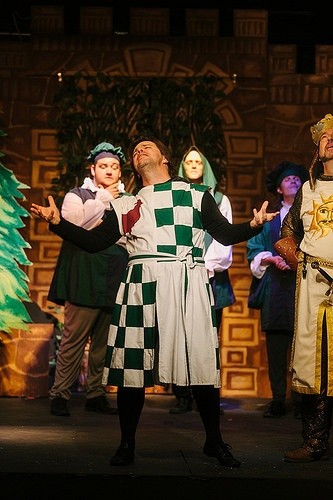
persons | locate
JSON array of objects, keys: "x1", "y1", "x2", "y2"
[
  {"x1": 246, "y1": 159, "x2": 309, "y2": 418},
  {"x1": 271, "y1": 113, "x2": 333, "y2": 463},
  {"x1": 49, "y1": 142, "x2": 120, "y2": 416},
  {"x1": 156, "y1": 146, "x2": 235, "y2": 417},
  {"x1": 32, "y1": 136, "x2": 282, "y2": 468}
]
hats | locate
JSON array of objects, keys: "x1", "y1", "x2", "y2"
[
  {"x1": 86, "y1": 141, "x2": 127, "y2": 166},
  {"x1": 309, "y1": 113, "x2": 333, "y2": 147},
  {"x1": 263, "y1": 160, "x2": 309, "y2": 198}
]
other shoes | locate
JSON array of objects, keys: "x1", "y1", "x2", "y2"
[
  {"x1": 110, "y1": 437, "x2": 135, "y2": 467},
  {"x1": 84, "y1": 395, "x2": 118, "y2": 415},
  {"x1": 52, "y1": 398, "x2": 72, "y2": 417},
  {"x1": 204, "y1": 442, "x2": 241, "y2": 468},
  {"x1": 288, "y1": 437, "x2": 329, "y2": 460},
  {"x1": 255, "y1": 399, "x2": 284, "y2": 411}
]
